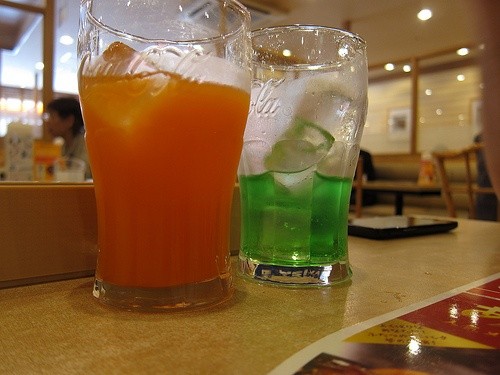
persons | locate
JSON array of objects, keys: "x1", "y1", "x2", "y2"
[
  {"x1": 350, "y1": 148, "x2": 379, "y2": 206},
  {"x1": 44, "y1": 98, "x2": 91, "y2": 178}
]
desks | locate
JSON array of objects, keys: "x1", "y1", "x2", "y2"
[
  {"x1": 0, "y1": 214, "x2": 500, "y2": 375},
  {"x1": 353, "y1": 181, "x2": 443, "y2": 216}
]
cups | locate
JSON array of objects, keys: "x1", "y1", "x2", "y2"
[
  {"x1": 236, "y1": 25, "x2": 369, "y2": 289},
  {"x1": 54, "y1": 157, "x2": 85, "y2": 182},
  {"x1": 76, "y1": 0, "x2": 253, "y2": 313}
]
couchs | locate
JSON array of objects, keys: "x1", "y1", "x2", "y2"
[{"x1": 372, "y1": 154, "x2": 477, "y2": 210}]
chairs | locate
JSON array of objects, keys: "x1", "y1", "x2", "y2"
[{"x1": 432, "y1": 144, "x2": 494, "y2": 220}]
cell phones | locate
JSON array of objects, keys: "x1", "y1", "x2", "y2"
[{"x1": 346, "y1": 217, "x2": 457, "y2": 240}]
